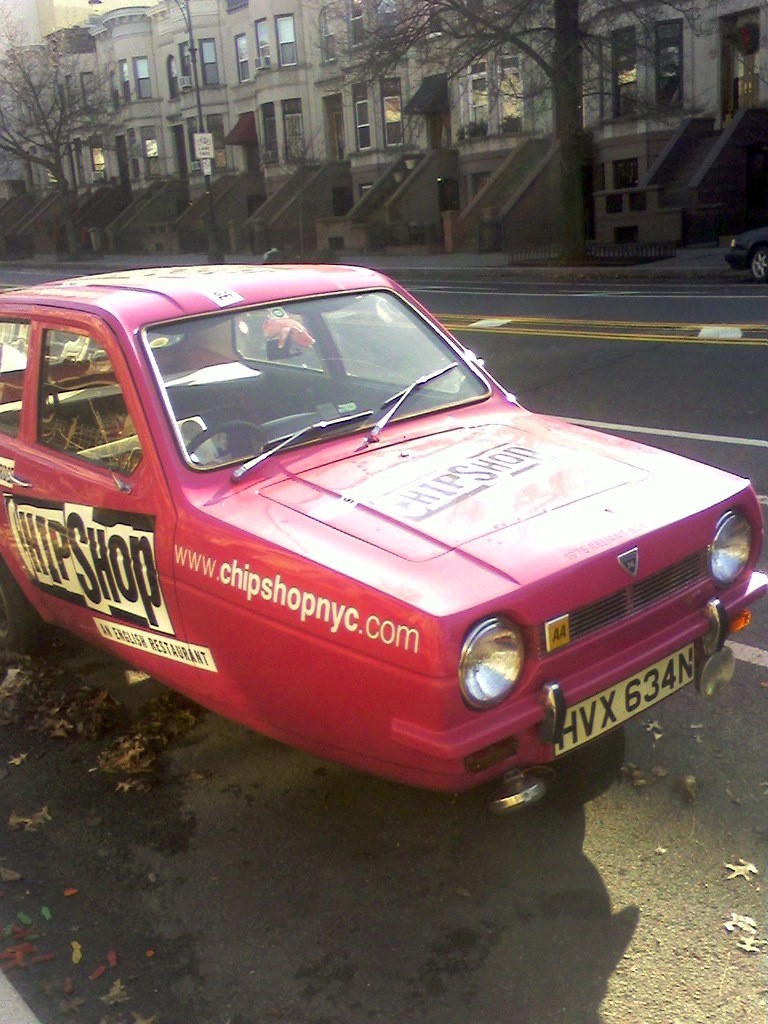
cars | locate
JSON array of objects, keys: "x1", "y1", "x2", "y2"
[
  {"x1": 0, "y1": 261, "x2": 768, "y2": 823},
  {"x1": 724, "y1": 227, "x2": 767, "y2": 280}
]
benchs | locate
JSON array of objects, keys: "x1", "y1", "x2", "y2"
[{"x1": 0, "y1": 376, "x2": 306, "y2": 455}]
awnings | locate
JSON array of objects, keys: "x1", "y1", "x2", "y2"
[
  {"x1": 402, "y1": 75, "x2": 448, "y2": 114},
  {"x1": 224, "y1": 112, "x2": 259, "y2": 144}
]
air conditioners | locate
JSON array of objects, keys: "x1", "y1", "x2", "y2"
[
  {"x1": 180, "y1": 76, "x2": 192, "y2": 88},
  {"x1": 191, "y1": 161, "x2": 201, "y2": 171},
  {"x1": 92, "y1": 171, "x2": 104, "y2": 181},
  {"x1": 262, "y1": 151, "x2": 278, "y2": 163},
  {"x1": 254, "y1": 57, "x2": 271, "y2": 70}
]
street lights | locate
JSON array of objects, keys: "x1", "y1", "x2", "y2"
[{"x1": 87, "y1": 0, "x2": 224, "y2": 265}]
